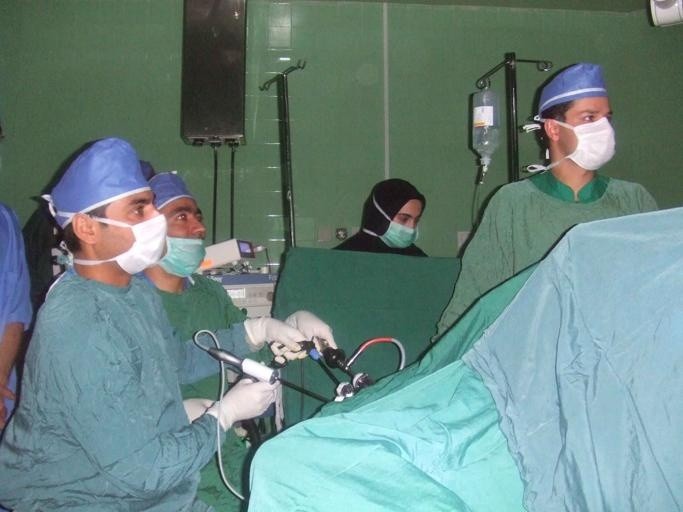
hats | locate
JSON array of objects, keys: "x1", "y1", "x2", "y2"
[
  {"x1": 538, "y1": 63, "x2": 608, "y2": 116},
  {"x1": 41, "y1": 137, "x2": 195, "y2": 230}
]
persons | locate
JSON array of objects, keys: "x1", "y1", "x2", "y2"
[
  {"x1": 430, "y1": 62, "x2": 660, "y2": 345},
  {"x1": 333, "y1": 178, "x2": 429, "y2": 256}
]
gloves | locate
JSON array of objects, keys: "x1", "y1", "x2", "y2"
[
  {"x1": 243, "y1": 310, "x2": 338, "y2": 364},
  {"x1": 183, "y1": 379, "x2": 280, "y2": 432}
]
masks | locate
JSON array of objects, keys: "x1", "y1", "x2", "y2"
[
  {"x1": 560, "y1": 117, "x2": 617, "y2": 172},
  {"x1": 100, "y1": 214, "x2": 168, "y2": 275},
  {"x1": 157, "y1": 236, "x2": 206, "y2": 278},
  {"x1": 379, "y1": 222, "x2": 418, "y2": 248}
]
